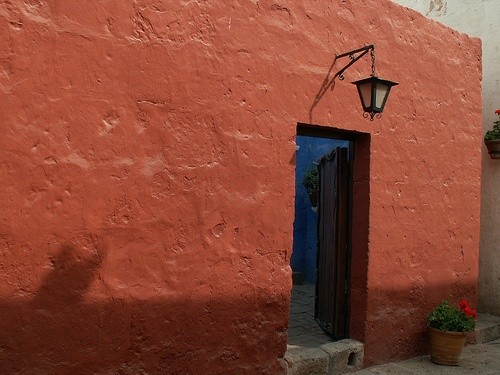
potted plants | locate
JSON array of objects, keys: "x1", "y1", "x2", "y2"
[{"x1": 302, "y1": 164, "x2": 318, "y2": 208}]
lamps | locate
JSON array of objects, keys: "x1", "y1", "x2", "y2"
[{"x1": 335, "y1": 44, "x2": 400, "y2": 121}]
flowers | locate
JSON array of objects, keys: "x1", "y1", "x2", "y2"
[
  {"x1": 426, "y1": 298, "x2": 477, "y2": 335},
  {"x1": 484, "y1": 109, "x2": 500, "y2": 139}
]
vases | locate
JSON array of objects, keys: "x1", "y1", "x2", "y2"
[
  {"x1": 484, "y1": 140, "x2": 500, "y2": 159},
  {"x1": 429, "y1": 326, "x2": 468, "y2": 366}
]
